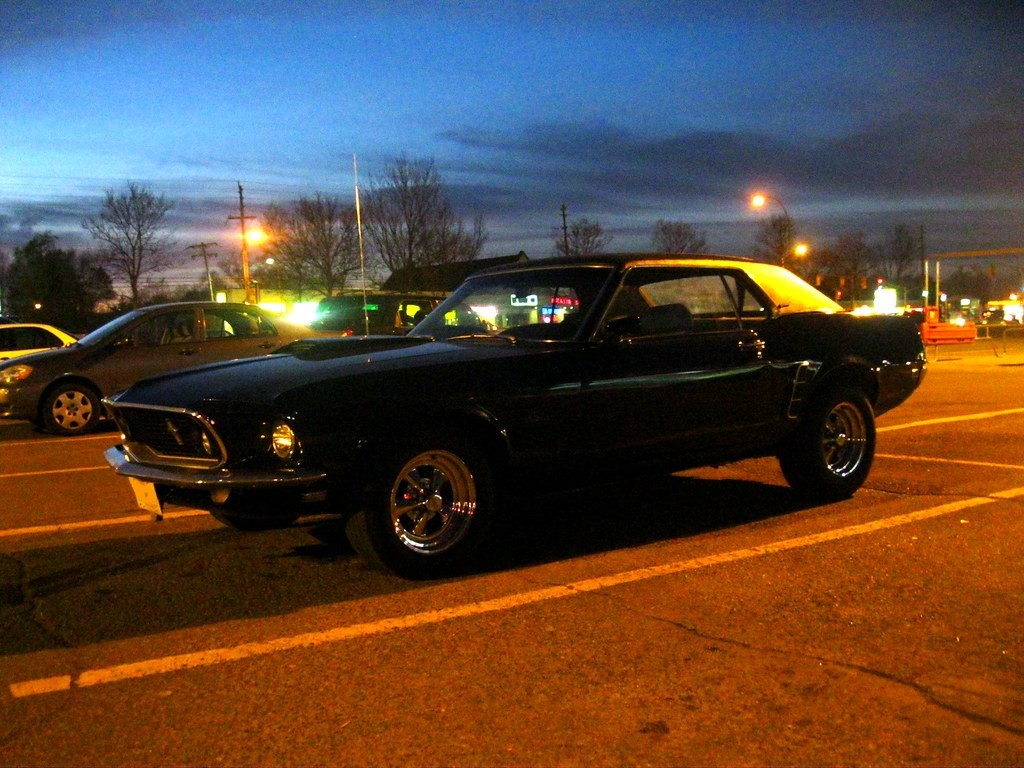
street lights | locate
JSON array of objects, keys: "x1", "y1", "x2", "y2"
[
  {"x1": 781, "y1": 244, "x2": 808, "y2": 268},
  {"x1": 751, "y1": 194, "x2": 791, "y2": 250}
]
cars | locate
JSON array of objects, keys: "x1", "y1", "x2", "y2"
[
  {"x1": 310, "y1": 290, "x2": 501, "y2": 335},
  {"x1": 0, "y1": 324, "x2": 79, "y2": 364},
  {"x1": 976, "y1": 304, "x2": 1024, "y2": 339},
  {"x1": 0, "y1": 301, "x2": 354, "y2": 437},
  {"x1": 98, "y1": 252, "x2": 929, "y2": 581}
]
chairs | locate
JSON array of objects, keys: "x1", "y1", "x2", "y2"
[
  {"x1": 171, "y1": 328, "x2": 184, "y2": 343},
  {"x1": 551, "y1": 313, "x2": 607, "y2": 342},
  {"x1": 640, "y1": 304, "x2": 694, "y2": 336},
  {"x1": 182, "y1": 325, "x2": 196, "y2": 342}
]
